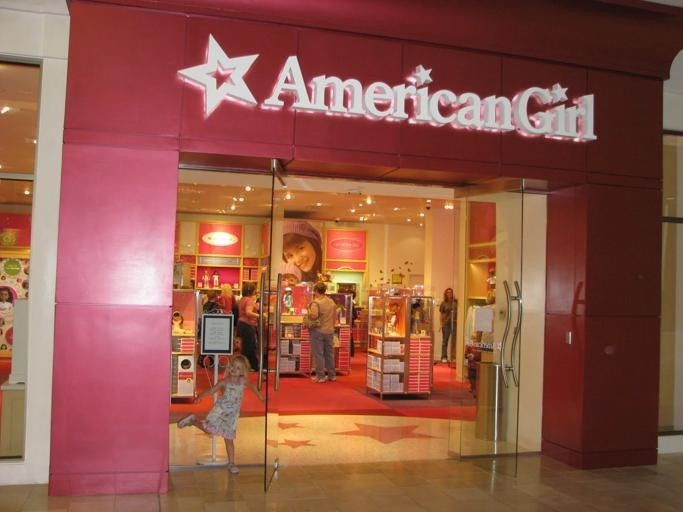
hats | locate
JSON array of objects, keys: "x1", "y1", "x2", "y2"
[{"x1": 282, "y1": 220, "x2": 322, "y2": 246}]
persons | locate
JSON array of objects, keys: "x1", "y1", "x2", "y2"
[
  {"x1": 217, "y1": 283, "x2": 236, "y2": 315},
  {"x1": 212, "y1": 271, "x2": 220, "y2": 288},
  {"x1": 338, "y1": 287, "x2": 357, "y2": 356},
  {"x1": 439, "y1": 288, "x2": 457, "y2": 363},
  {"x1": 171, "y1": 311, "x2": 185, "y2": 335},
  {"x1": 0, "y1": 288, "x2": 12, "y2": 312},
  {"x1": 282, "y1": 220, "x2": 322, "y2": 283},
  {"x1": 464, "y1": 332, "x2": 482, "y2": 398},
  {"x1": 304, "y1": 283, "x2": 337, "y2": 384},
  {"x1": 411, "y1": 301, "x2": 422, "y2": 334},
  {"x1": 177, "y1": 355, "x2": 265, "y2": 474},
  {"x1": 236, "y1": 281, "x2": 260, "y2": 373},
  {"x1": 202, "y1": 290, "x2": 223, "y2": 314},
  {"x1": 202, "y1": 270, "x2": 210, "y2": 289},
  {"x1": 283, "y1": 287, "x2": 294, "y2": 313}
]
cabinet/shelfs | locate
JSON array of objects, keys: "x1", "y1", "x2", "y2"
[
  {"x1": 456, "y1": 201, "x2": 495, "y2": 384},
  {"x1": 368, "y1": 294, "x2": 435, "y2": 402},
  {"x1": 171, "y1": 288, "x2": 202, "y2": 402},
  {"x1": 267, "y1": 289, "x2": 353, "y2": 376},
  {"x1": 0, "y1": 299, "x2": 28, "y2": 458},
  {"x1": 175, "y1": 221, "x2": 270, "y2": 338}
]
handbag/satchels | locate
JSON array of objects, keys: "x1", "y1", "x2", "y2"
[{"x1": 302, "y1": 301, "x2": 321, "y2": 329}]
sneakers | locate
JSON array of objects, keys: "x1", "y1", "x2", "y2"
[
  {"x1": 177, "y1": 413, "x2": 197, "y2": 429},
  {"x1": 311, "y1": 375, "x2": 337, "y2": 383},
  {"x1": 440, "y1": 357, "x2": 456, "y2": 363},
  {"x1": 227, "y1": 462, "x2": 240, "y2": 474}
]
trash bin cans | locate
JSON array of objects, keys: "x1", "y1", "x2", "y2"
[{"x1": 476, "y1": 362, "x2": 508, "y2": 442}]
